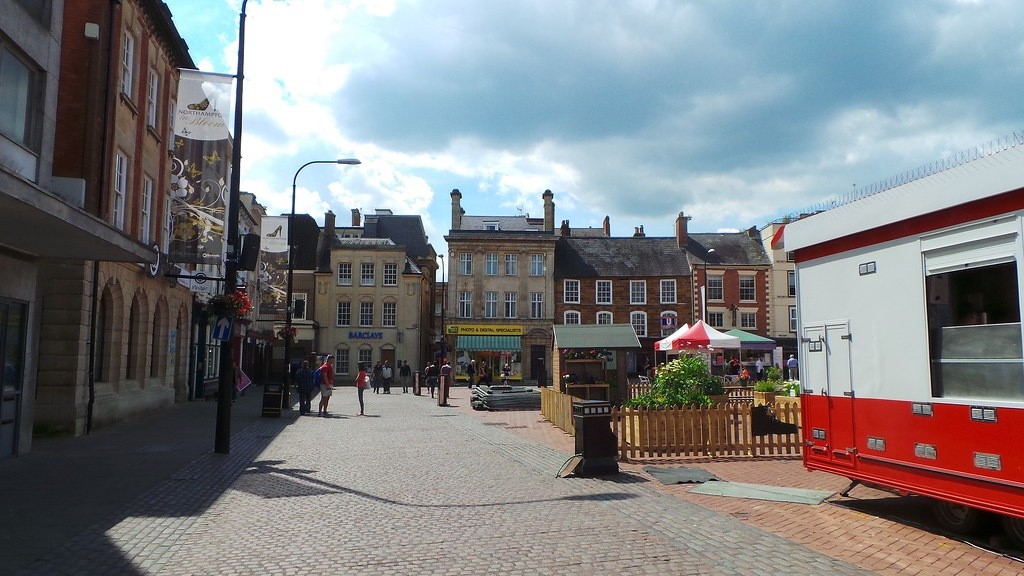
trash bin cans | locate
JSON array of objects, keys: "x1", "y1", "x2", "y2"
[
  {"x1": 262, "y1": 383, "x2": 284, "y2": 417},
  {"x1": 571, "y1": 399, "x2": 619, "y2": 478}
]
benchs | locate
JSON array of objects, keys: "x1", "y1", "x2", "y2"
[{"x1": 728, "y1": 396, "x2": 754, "y2": 400}]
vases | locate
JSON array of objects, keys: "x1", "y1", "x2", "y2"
[
  {"x1": 213, "y1": 303, "x2": 233, "y2": 316},
  {"x1": 741, "y1": 379, "x2": 747, "y2": 387}
]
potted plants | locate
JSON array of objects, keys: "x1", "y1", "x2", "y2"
[
  {"x1": 625, "y1": 354, "x2": 730, "y2": 452},
  {"x1": 754, "y1": 366, "x2": 800, "y2": 407}
]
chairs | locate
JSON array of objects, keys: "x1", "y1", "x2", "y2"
[{"x1": 638, "y1": 375, "x2": 650, "y2": 384}]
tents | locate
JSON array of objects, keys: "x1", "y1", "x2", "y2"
[
  {"x1": 724, "y1": 328, "x2": 776, "y2": 368},
  {"x1": 654, "y1": 319, "x2": 742, "y2": 386}
]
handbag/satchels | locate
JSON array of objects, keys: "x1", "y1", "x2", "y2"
[{"x1": 364, "y1": 377, "x2": 371, "y2": 389}]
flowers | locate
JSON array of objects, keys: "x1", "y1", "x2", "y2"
[
  {"x1": 562, "y1": 370, "x2": 605, "y2": 384},
  {"x1": 737, "y1": 369, "x2": 750, "y2": 383},
  {"x1": 277, "y1": 325, "x2": 298, "y2": 344},
  {"x1": 563, "y1": 349, "x2": 598, "y2": 360},
  {"x1": 210, "y1": 292, "x2": 254, "y2": 317}
]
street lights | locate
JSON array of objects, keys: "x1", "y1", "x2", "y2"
[
  {"x1": 438, "y1": 253, "x2": 444, "y2": 367},
  {"x1": 281, "y1": 158, "x2": 361, "y2": 409},
  {"x1": 705, "y1": 249, "x2": 715, "y2": 324}
]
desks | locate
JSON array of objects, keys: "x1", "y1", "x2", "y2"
[{"x1": 722, "y1": 386, "x2": 754, "y2": 395}]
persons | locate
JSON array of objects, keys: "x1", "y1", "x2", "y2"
[
  {"x1": 355, "y1": 361, "x2": 366, "y2": 416},
  {"x1": 466, "y1": 360, "x2": 476, "y2": 389},
  {"x1": 371, "y1": 360, "x2": 392, "y2": 394},
  {"x1": 318, "y1": 355, "x2": 335, "y2": 417},
  {"x1": 501, "y1": 363, "x2": 510, "y2": 385},
  {"x1": 296, "y1": 360, "x2": 314, "y2": 415},
  {"x1": 649, "y1": 361, "x2": 661, "y2": 384},
  {"x1": 476, "y1": 361, "x2": 492, "y2": 387},
  {"x1": 425, "y1": 359, "x2": 452, "y2": 398},
  {"x1": 400, "y1": 360, "x2": 411, "y2": 393},
  {"x1": 729, "y1": 354, "x2": 797, "y2": 380}
]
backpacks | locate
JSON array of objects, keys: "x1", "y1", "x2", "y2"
[{"x1": 313, "y1": 367, "x2": 322, "y2": 389}]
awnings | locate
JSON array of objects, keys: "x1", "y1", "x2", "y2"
[{"x1": 457, "y1": 336, "x2": 522, "y2": 353}]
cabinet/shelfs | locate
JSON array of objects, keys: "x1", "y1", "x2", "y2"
[{"x1": 564, "y1": 359, "x2": 610, "y2": 403}]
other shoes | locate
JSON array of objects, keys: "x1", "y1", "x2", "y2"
[
  {"x1": 318, "y1": 413, "x2": 333, "y2": 417},
  {"x1": 356, "y1": 413, "x2": 364, "y2": 415}
]
